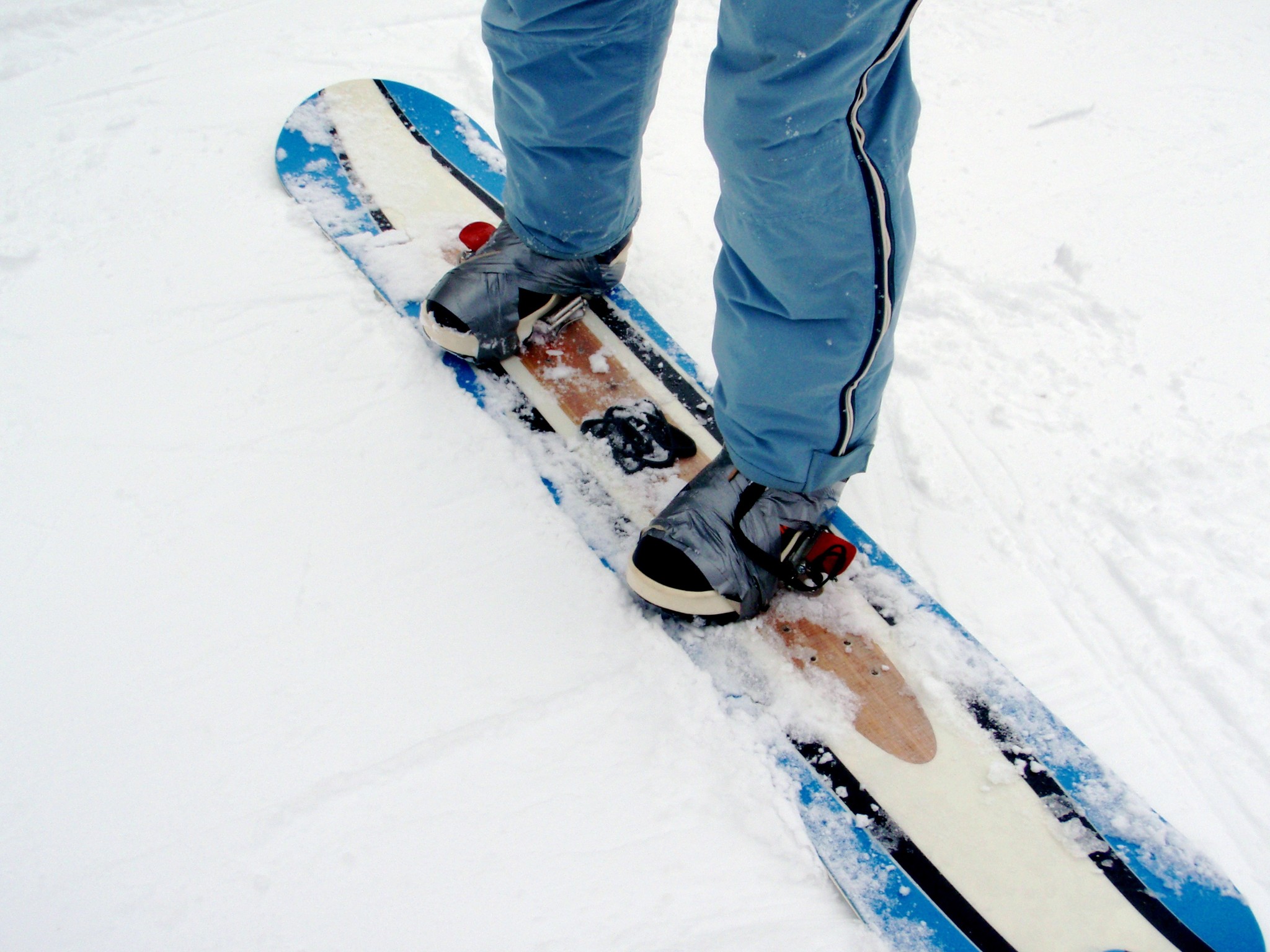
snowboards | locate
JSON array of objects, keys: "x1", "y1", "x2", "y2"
[{"x1": 276, "y1": 79, "x2": 1267, "y2": 952}]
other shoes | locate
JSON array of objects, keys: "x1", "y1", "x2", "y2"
[
  {"x1": 625, "y1": 435, "x2": 852, "y2": 615},
  {"x1": 419, "y1": 223, "x2": 636, "y2": 358}
]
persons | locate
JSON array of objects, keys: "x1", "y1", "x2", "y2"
[{"x1": 418, "y1": 0, "x2": 927, "y2": 626}]
